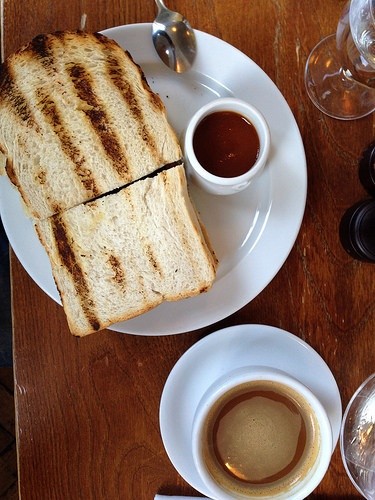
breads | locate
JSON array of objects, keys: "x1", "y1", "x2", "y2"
[
  {"x1": 35, "y1": 163, "x2": 220, "y2": 337},
  {"x1": 0, "y1": 27, "x2": 183, "y2": 224}
]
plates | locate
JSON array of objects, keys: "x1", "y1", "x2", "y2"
[
  {"x1": 159, "y1": 324, "x2": 342, "y2": 500},
  {"x1": 339, "y1": 373, "x2": 375, "y2": 500},
  {"x1": 0, "y1": 23, "x2": 308, "y2": 336}
]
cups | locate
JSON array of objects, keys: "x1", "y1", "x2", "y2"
[
  {"x1": 184, "y1": 97, "x2": 271, "y2": 196},
  {"x1": 192, "y1": 373, "x2": 332, "y2": 500}
]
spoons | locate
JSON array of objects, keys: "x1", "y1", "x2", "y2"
[{"x1": 151, "y1": 0, "x2": 197, "y2": 74}]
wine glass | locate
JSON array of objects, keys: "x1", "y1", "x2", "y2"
[{"x1": 304, "y1": 0, "x2": 375, "y2": 120}]
